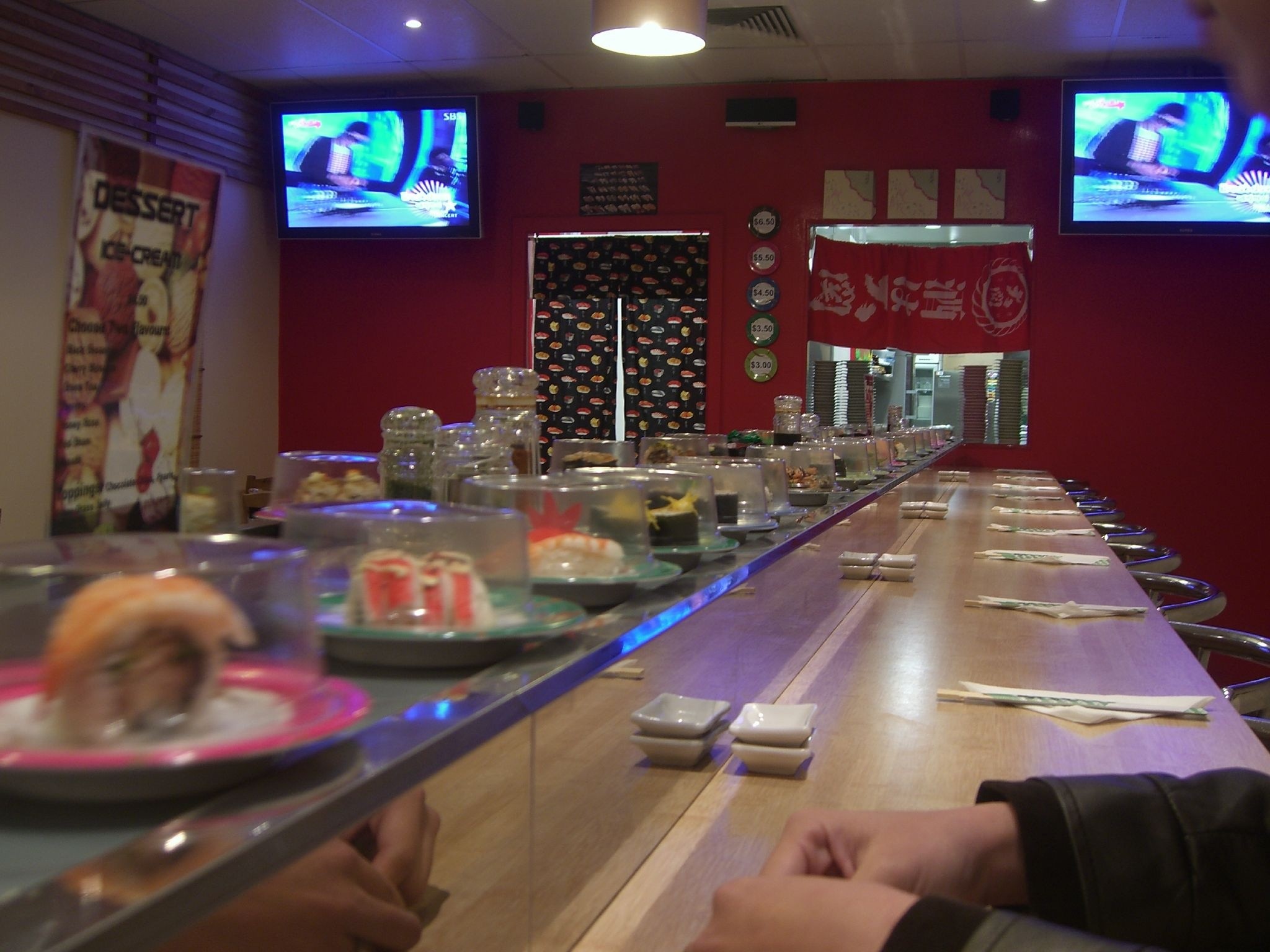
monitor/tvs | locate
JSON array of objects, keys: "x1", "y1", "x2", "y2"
[
  {"x1": 267, "y1": 93, "x2": 482, "y2": 242},
  {"x1": 1059, "y1": 79, "x2": 1270, "y2": 237}
]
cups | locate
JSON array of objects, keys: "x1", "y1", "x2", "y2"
[{"x1": 177, "y1": 465, "x2": 243, "y2": 529}]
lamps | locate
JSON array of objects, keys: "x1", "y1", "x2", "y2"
[{"x1": 588, "y1": 0, "x2": 709, "y2": 60}]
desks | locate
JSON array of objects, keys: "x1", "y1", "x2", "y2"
[{"x1": 552, "y1": 463, "x2": 1269, "y2": 952}]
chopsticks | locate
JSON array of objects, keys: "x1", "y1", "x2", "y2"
[{"x1": 936, "y1": 469, "x2": 1216, "y2": 708}]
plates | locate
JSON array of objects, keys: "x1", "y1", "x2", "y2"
[
  {"x1": 480, "y1": 563, "x2": 683, "y2": 606},
  {"x1": 1100, "y1": 183, "x2": 1190, "y2": 200},
  {"x1": 878, "y1": 553, "x2": 917, "y2": 581},
  {"x1": 813, "y1": 360, "x2": 876, "y2": 433},
  {"x1": 305, "y1": 193, "x2": 375, "y2": 210},
  {"x1": 716, "y1": 437, "x2": 951, "y2": 545},
  {"x1": 925, "y1": 471, "x2": 971, "y2": 518},
  {"x1": 727, "y1": 702, "x2": 820, "y2": 777},
  {"x1": 317, "y1": 592, "x2": 589, "y2": 668},
  {"x1": 837, "y1": 550, "x2": 878, "y2": 581},
  {"x1": 628, "y1": 693, "x2": 730, "y2": 768},
  {"x1": 0, "y1": 658, "x2": 373, "y2": 804},
  {"x1": 901, "y1": 470, "x2": 954, "y2": 518},
  {"x1": 958, "y1": 359, "x2": 1028, "y2": 445},
  {"x1": 575, "y1": 527, "x2": 741, "y2": 572},
  {"x1": 254, "y1": 506, "x2": 296, "y2": 525}
]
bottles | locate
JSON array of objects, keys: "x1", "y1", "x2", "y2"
[
  {"x1": 380, "y1": 366, "x2": 544, "y2": 510},
  {"x1": 772, "y1": 393, "x2": 909, "y2": 445}
]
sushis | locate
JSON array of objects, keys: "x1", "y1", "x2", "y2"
[
  {"x1": 291, "y1": 438, "x2": 906, "y2": 633},
  {"x1": 36, "y1": 571, "x2": 256, "y2": 750}
]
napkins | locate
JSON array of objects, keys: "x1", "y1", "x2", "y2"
[
  {"x1": 976, "y1": 594, "x2": 1149, "y2": 621},
  {"x1": 991, "y1": 523, "x2": 1096, "y2": 538},
  {"x1": 993, "y1": 468, "x2": 1048, "y2": 475},
  {"x1": 990, "y1": 482, "x2": 1062, "y2": 493},
  {"x1": 987, "y1": 492, "x2": 1064, "y2": 502},
  {"x1": 993, "y1": 474, "x2": 1057, "y2": 481},
  {"x1": 984, "y1": 547, "x2": 1113, "y2": 569},
  {"x1": 958, "y1": 680, "x2": 1219, "y2": 728},
  {"x1": 992, "y1": 504, "x2": 1080, "y2": 517}
]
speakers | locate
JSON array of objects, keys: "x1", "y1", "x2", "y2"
[
  {"x1": 517, "y1": 101, "x2": 546, "y2": 131},
  {"x1": 989, "y1": 89, "x2": 1019, "y2": 120}
]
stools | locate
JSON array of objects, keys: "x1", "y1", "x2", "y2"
[{"x1": 1056, "y1": 478, "x2": 1270, "y2": 746}]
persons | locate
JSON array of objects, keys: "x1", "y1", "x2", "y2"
[
  {"x1": 691, "y1": 767, "x2": 1270, "y2": 952},
  {"x1": 298, "y1": 123, "x2": 465, "y2": 201},
  {"x1": 146, "y1": 776, "x2": 448, "y2": 952},
  {"x1": 1091, "y1": 102, "x2": 1190, "y2": 181}
]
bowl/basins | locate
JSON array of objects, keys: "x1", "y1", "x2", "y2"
[{"x1": 727, "y1": 443, "x2": 746, "y2": 456}]
